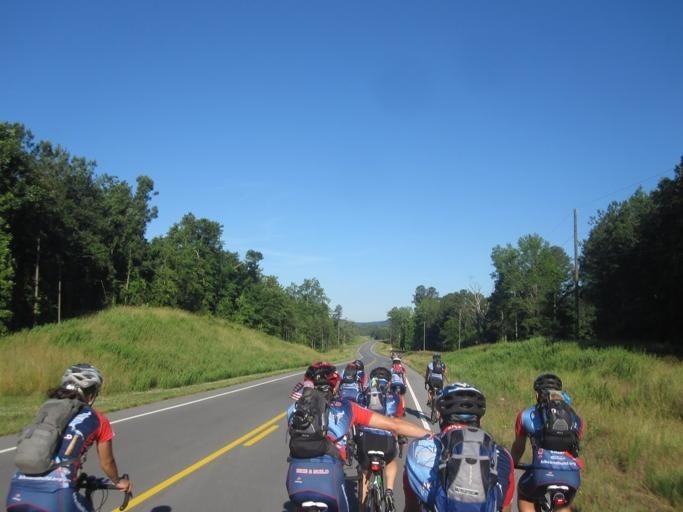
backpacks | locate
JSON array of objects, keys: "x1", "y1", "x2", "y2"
[
  {"x1": 537, "y1": 399, "x2": 579, "y2": 458},
  {"x1": 13, "y1": 398, "x2": 91, "y2": 476},
  {"x1": 362, "y1": 388, "x2": 389, "y2": 416},
  {"x1": 354, "y1": 360, "x2": 364, "y2": 370},
  {"x1": 433, "y1": 360, "x2": 442, "y2": 373},
  {"x1": 392, "y1": 364, "x2": 404, "y2": 375},
  {"x1": 286, "y1": 386, "x2": 343, "y2": 462},
  {"x1": 434, "y1": 427, "x2": 497, "y2": 512},
  {"x1": 341, "y1": 369, "x2": 360, "y2": 385}
]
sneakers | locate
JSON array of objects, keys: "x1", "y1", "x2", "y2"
[{"x1": 384, "y1": 490, "x2": 396, "y2": 512}]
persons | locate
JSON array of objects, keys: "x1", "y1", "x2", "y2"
[
  {"x1": 7, "y1": 364, "x2": 133, "y2": 512},
  {"x1": 287, "y1": 354, "x2": 439, "y2": 512},
  {"x1": 403, "y1": 382, "x2": 515, "y2": 512},
  {"x1": 511, "y1": 373, "x2": 585, "y2": 512},
  {"x1": 425, "y1": 353, "x2": 447, "y2": 405}
]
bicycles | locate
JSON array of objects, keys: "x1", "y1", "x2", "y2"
[
  {"x1": 79, "y1": 473, "x2": 132, "y2": 510},
  {"x1": 425, "y1": 382, "x2": 440, "y2": 422},
  {"x1": 516, "y1": 464, "x2": 569, "y2": 512},
  {"x1": 302, "y1": 448, "x2": 395, "y2": 512}
]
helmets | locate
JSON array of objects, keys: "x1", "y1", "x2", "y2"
[
  {"x1": 370, "y1": 367, "x2": 392, "y2": 382},
  {"x1": 347, "y1": 363, "x2": 356, "y2": 368},
  {"x1": 60, "y1": 363, "x2": 103, "y2": 393},
  {"x1": 533, "y1": 373, "x2": 562, "y2": 392},
  {"x1": 304, "y1": 360, "x2": 340, "y2": 386},
  {"x1": 433, "y1": 352, "x2": 442, "y2": 359},
  {"x1": 435, "y1": 381, "x2": 485, "y2": 416},
  {"x1": 393, "y1": 357, "x2": 401, "y2": 363}
]
